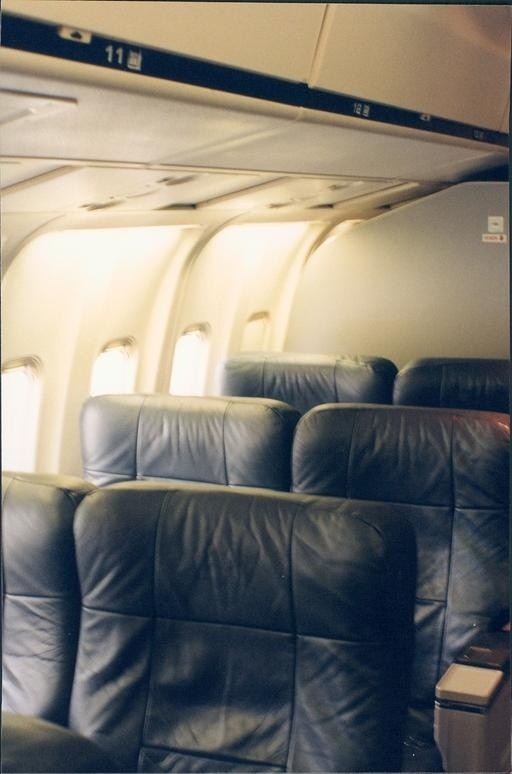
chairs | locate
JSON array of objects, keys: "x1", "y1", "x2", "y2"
[
  {"x1": 1, "y1": 469, "x2": 96, "y2": 728},
  {"x1": 288, "y1": 402, "x2": 510, "y2": 772},
  {"x1": 216, "y1": 357, "x2": 398, "y2": 415},
  {"x1": 78, "y1": 391, "x2": 295, "y2": 491},
  {"x1": 69, "y1": 478, "x2": 419, "y2": 773},
  {"x1": 392, "y1": 356, "x2": 511, "y2": 414}
]
